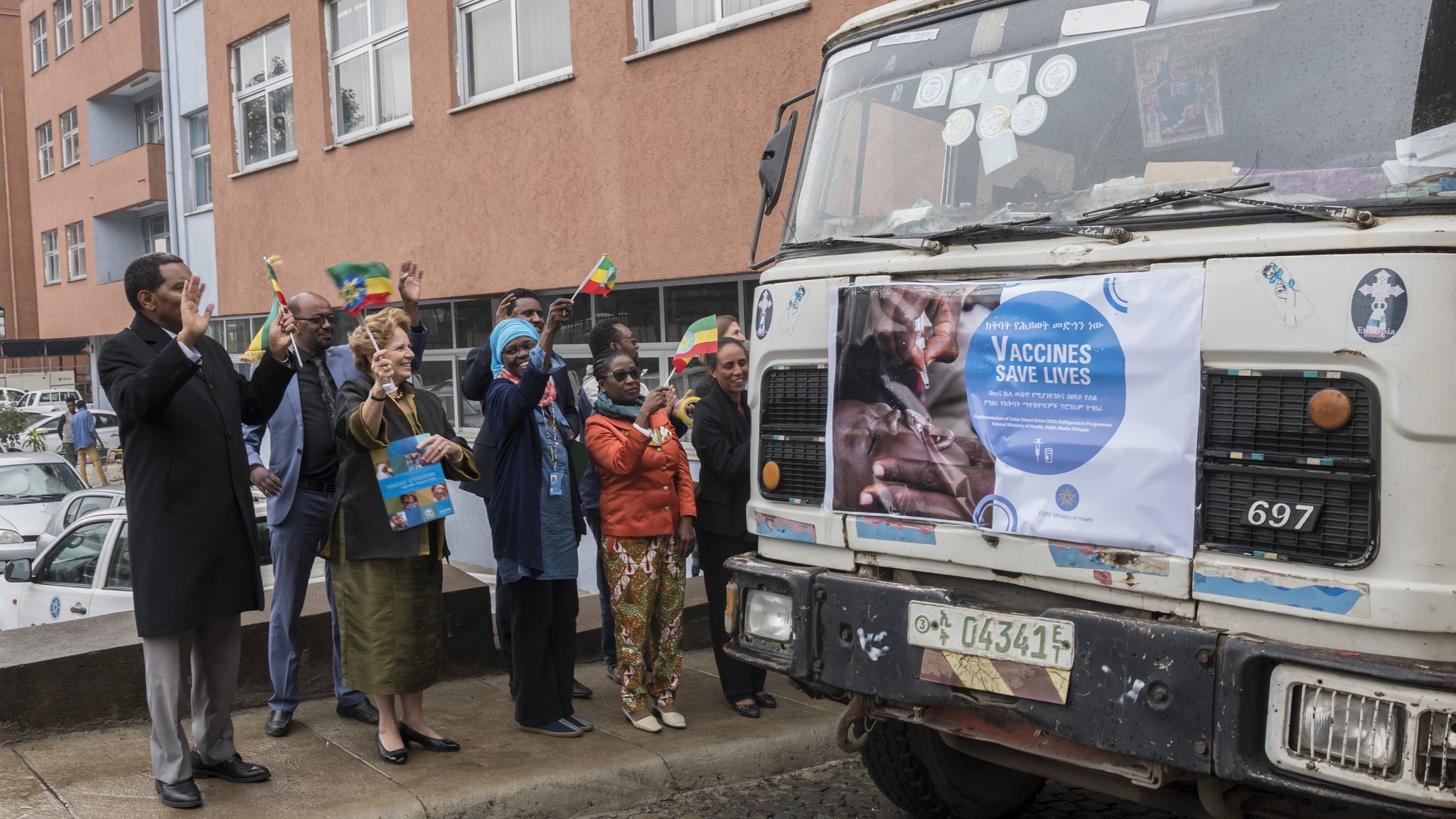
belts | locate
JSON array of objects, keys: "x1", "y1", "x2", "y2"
[{"x1": 297, "y1": 478, "x2": 335, "y2": 494}]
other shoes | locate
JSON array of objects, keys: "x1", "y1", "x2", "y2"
[
  {"x1": 398, "y1": 720, "x2": 460, "y2": 752},
  {"x1": 651, "y1": 701, "x2": 687, "y2": 728},
  {"x1": 733, "y1": 703, "x2": 760, "y2": 717},
  {"x1": 753, "y1": 693, "x2": 777, "y2": 708},
  {"x1": 375, "y1": 729, "x2": 408, "y2": 764},
  {"x1": 623, "y1": 707, "x2": 662, "y2": 732},
  {"x1": 606, "y1": 666, "x2": 622, "y2": 684}
]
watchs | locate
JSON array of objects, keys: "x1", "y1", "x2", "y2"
[
  {"x1": 274, "y1": 350, "x2": 289, "y2": 362},
  {"x1": 369, "y1": 388, "x2": 387, "y2": 402}
]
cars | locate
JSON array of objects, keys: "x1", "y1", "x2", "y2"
[{"x1": 0, "y1": 366, "x2": 739, "y2": 632}]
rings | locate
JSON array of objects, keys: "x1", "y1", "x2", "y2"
[
  {"x1": 563, "y1": 310, "x2": 566, "y2": 317},
  {"x1": 411, "y1": 277, "x2": 415, "y2": 279},
  {"x1": 441, "y1": 451, "x2": 446, "y2": 454},
  {"x1": 436, "y1": 447, "x2": 441, "y2": 451},
  {"x1": 383, "y1": 366, "x2": 387, "y2": 372}
]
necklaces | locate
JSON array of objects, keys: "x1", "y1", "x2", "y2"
[{"x1": 391, "y1": 388, "x2": 404, "y2": 400}]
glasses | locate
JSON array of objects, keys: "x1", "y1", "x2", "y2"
[
  {"x1": 295, "y1": 316, "x2": 338, "y2": 325},
  {"x1": 609, "y1": 338, "x2": 636, "y2": 349},
  {"x1": 502, "y1": 340, "x2": 537, "y2": 358},
  {"x1": 601, "y1": 367, "x2": 643, "y2": 382}
]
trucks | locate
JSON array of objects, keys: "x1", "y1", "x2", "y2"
[{"x1": 721, "y1": 0, "x2": 1456, "y2": 819}]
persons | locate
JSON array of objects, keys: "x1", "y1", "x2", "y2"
[
  {"x1": 241, "y1": 262, "x2": 460, "y2": 763},
  {"x1": 459, "y1": 288, "x2": 758, "y2": 737},
  {"x1": 96, "y1": 253, "x2": 298, "y2": 811},
  {"x1": 57, "y1": 397, "x2": 111, "y2": 488},
  {"x1": 832, "y1": 281, "x2": 996, "y2": 529}
]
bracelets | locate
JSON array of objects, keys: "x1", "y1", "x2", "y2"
[{"x1": 446, "y1": 444, "x2": 464, "y2": 463}]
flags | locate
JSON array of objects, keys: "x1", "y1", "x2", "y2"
[
  {"x1": 238, "y1": 254, "x2": 288, "y2": 364},
  {"x1": 327, "y1": 261, "x2": 398, "y2": 318},
  {"x1": 582, "y1": 257, "x2": 617, "y2": 299},
  {"x1": 672, "y1": 314, "x2": 718, "y2": 375}
]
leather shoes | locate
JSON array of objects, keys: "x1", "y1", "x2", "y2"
[
  {"x1": 264, "y1": 709, "x2": 293, "y2": 737},
  {"x1": 155, "y1": 777, "x2": 203, "y2": 807},
  {"x1": 572, "y1": 678, "x2": 593, "y2": 696},
  {"x1": 190, "y1": 747, "x2": 272, "y2": 782},
  {"x1": 336, "y1": 697, "x2": 380, "y2": 724}
]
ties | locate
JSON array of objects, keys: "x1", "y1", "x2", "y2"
[{"x1": 309, "y1": 357, "x2": 337, "y2": 441}]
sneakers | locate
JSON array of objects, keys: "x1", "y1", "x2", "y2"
[
  {"x1": 519, "y1": 717, "x2": 584, "y2": 737},
  {"x1": 564, "y1": 714, "x2": 594, "y2": 731}
]
